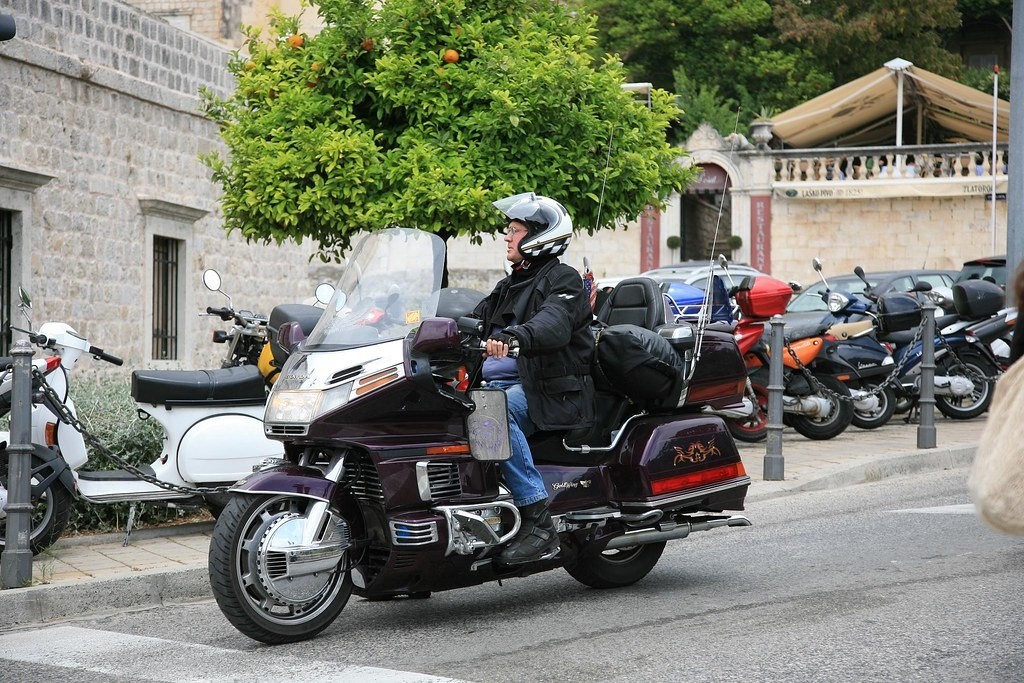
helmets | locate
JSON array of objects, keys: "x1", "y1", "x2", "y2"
[{"x1": 506, "y1": 196, "x2": 573, "y2": 259}]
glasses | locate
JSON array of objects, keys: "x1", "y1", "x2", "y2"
[{"x1": 504, "y1": 227, "x2": 529, "y2": 236}]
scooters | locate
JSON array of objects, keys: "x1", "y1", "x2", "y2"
[{"x1": 0, "y1": 284, "x2": 302, "y2": 558}]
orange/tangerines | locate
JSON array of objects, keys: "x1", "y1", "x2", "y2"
[{"x1": 243, "y1": 26, "x2": 464, "y2": 98}]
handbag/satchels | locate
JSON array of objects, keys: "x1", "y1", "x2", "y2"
[
  {"x1": 965, "y1": 356, "x2": 1024, "y2": 535},
  {"x1": 589, "y1": 319, "x2": 684, "y2": 415}
]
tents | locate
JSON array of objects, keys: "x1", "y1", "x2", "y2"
[{"x1": 768, "y1": 57, "x2": 1010, "y2": 178}]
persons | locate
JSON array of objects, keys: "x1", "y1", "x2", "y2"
[
  {"x1": 451, "y1": 190, "x2": 594, "y2": 564},
  {"x1": 834, "y1": 153, "x2": 987, "y2": 178}
]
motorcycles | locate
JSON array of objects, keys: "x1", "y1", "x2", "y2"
[
  {"x1": 204, "y1": 224, "x2": 758, "y2": 646},
  {"x1": 196, "y1": 266, "x2": 1024, "y2": 444}
]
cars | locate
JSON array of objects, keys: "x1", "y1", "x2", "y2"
[
  {"x1": 787, "y1": 268, "x2": 963, "y2": 322},
  {"x1": 634, "y1": 259, "x2": 772, "y2": 311},
  {"x1": 951, "y1": 253, "x2": 1010, "y2": 299}
]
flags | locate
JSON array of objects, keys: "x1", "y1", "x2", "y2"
[{"x1": 583, "y1": 272, "x2": 597, "y2": 309}]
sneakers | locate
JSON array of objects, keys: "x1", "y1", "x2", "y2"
[{"x1": 499, "y1": 507, "x2": 561, "y2": 565}]
